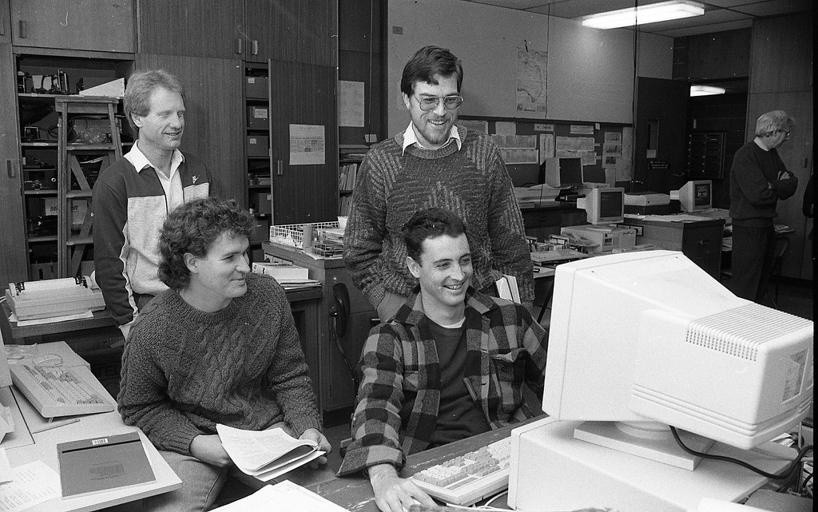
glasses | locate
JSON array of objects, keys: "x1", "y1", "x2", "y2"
[{"x1": 410, "y1": 92, "x2": 463, "y2": 113}]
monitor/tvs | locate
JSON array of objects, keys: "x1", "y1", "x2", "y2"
[
  {"x1": 576, "y1": 187, "x2": 624, "y2": 231},
  {"x1": 540, "y1": 249, "x2": 815, "y2": 473},
  {"x1": 546, "y1": 156, "x2": 583, "y2": 188},
  {"x1": 670, "y1": 180, "x2": 712, "y2": 215},
  {"x1": 0, "y1": 326, "x2": 14, "y2": 389}
]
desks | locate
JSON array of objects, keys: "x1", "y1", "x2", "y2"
[
  {"x1": 2, "y1": 274, "x2": 320, "y2": 430},
  {"x1": 522, "y1": 262, "x2": 555, "y2": 328},
  {"x1": 213, "y1": 412, "x2": 548, "y2": 511}
]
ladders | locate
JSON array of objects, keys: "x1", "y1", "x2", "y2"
[{"x1": 56, "y1": 95, "x2": 124, "y2": 279}]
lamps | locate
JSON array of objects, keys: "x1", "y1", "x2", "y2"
[{"x1": 581, "y1": 0, "x2": 706, "y2": 31}]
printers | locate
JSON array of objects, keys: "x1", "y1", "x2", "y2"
[
  {"x1": 4, "y1": 275, "x2": 114, "y2": 321},
  {"x1": 624, "y1": 189, "x2": 670, "y2": 216}
]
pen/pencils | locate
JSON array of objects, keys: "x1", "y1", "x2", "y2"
[{"x1": 31, "y1": 418, "x2": 80, "y2": 434}]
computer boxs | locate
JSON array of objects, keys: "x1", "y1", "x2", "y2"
[
  {"x1": 560, "y1": 225, "x2": 636, "y2": 252},
  {"x1": 507, "y1": 416, "x2": 799, "y2": 509}
]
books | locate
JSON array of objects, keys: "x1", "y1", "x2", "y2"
[
  {"x1": 214, "y1": 419, "x2": 324, "y2": 483},
  {"x1": 501, "y1": 271, "x2": 522, "y2": 307},
  {"x1": 492, "y1": 275, "x2": 513, "y2": 304},
  {"x1": 276, "y1": 278, "x2": 321, "y2": 291}
]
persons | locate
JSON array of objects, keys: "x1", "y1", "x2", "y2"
[
  {"x1": 91, "y1": 68, "x2": 226, "y2": 342},
  {"x1": 332, "y1": 206, "x2": 549, "y2": 511},
  {"x1": 342, "y1": 43, "x2": 536, "y2": 324},
  {"x1": 728, "y1": 109, "x2": 796, "y2": 310},
  {"x1": 802, "y1": 174, "x2": 817, "y2": 258},
  {"x1": 117, "y1": 195, "x2": 333, "y2": 511}
]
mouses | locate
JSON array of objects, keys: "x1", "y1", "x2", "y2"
[
  {"x1": 32, "y1": 353, "x2": 63, "y2": 367},
  {"x1": 400, "y1": 498, "x2": 425, "y2": 512}
]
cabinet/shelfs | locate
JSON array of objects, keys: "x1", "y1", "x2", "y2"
[
  {"x1": 745, "y1": 10, "x2": 816, "y2": 91},
  {"x1": 745, "y1": 91, "x2": 817, "y2": 282},
  {"x1": 1, "y1": 53, "x2": 136, "y2": 283},
  {"x1": 136, "y1": 53, "x2": 339, "y2": 228},
  {"x1": 137, "y1": 1, "x2": 337, "y2": 66},
  {"x1": 262, "y1": 237, "x2": 381, "y2": 429},
  {"x1": 630, "y1": 211, "x2": 724, "y2": 286},
  {"x1": 7, "y1": 1, "x2": 136, "y2": 55}
]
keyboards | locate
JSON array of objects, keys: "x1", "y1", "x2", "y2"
[
  {"x1": 9, "y1": 365, "x2": 114, "y2": 423},
  {"x1": 405, "y1": 435, "x2": 529, "y2": 506}
]
